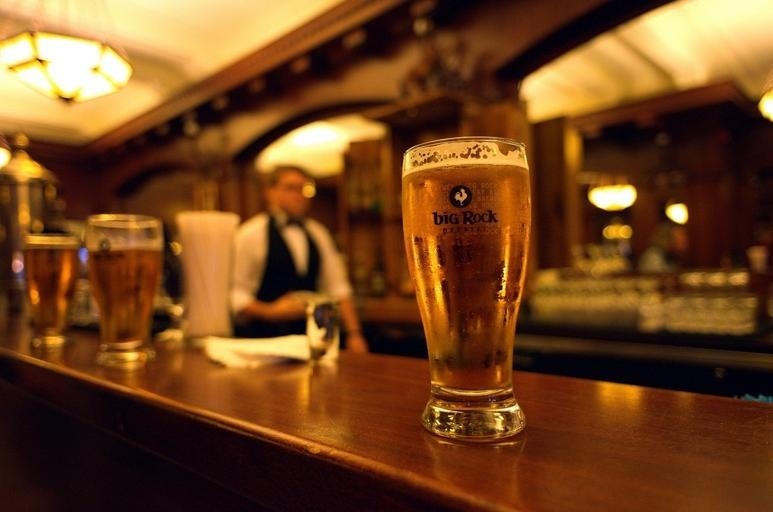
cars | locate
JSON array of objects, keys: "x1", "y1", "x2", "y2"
[
  {"x1": 23, "y1": 210, "x2": 245, "y2": 368},
  {"x1": 402, "y1": 136, "x2": 533, "y2": 442}
]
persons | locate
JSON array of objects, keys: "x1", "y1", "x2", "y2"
[{"x1": 230, "y1": 166, "x2": 370, "y2": 356}]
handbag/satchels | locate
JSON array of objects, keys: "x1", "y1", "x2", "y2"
[{"x1": 342, "y1": 327, "x2": 363, "y2": 333}]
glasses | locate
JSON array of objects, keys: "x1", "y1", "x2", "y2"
[{"x1": 1, "y1": 31, "x2": 134, "y2": 105}]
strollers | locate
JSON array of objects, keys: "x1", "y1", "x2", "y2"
[{"x1": 286, "y1": 217, "x2": 303, "y2": 227}]
